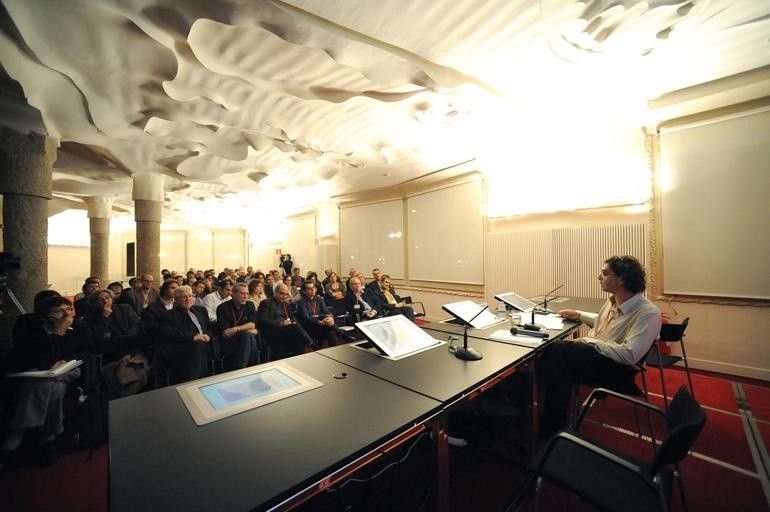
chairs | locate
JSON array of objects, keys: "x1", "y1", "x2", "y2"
[
  {"x1": 564, "y1": 330, "x2": 661, "y2": 454},
  {"x1": 645, "y1": 312, "x2": 698, "y2": 406},
  {"x1": 532, "y1": 380, "x2": 706, "y2": 511},
  {"x1": 3, "y1": 311, "x2": 97, "y2": 460},
  {"x1": 99, "y1": 301, "x2": 427, "y2": 400}
]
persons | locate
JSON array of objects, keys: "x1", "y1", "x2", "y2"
[
  {"x1": 536, "y1": 255, "x2": 663, "y2": 441},
  {"x1": 0, "y1": 265, "x2": 414, "y2": 463},
  {"x1": 282, "y1": 254, "x2": 293, "y2": 275}
]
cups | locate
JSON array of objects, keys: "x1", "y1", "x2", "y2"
[{"x1": 511, "y1": 309, "x2": 521, "y2": 326}]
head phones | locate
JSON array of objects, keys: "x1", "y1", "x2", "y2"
[{"x1": 289, "y1": 254, "x2": 292, "y2": 259}]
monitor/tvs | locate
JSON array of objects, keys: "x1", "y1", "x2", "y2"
[
  {"x1": 494, "y1": 291, "x2": 540, "y2": 313},
  {"x1": 354, "y1": 314, "x2": 441, "y2": 359},
  {"x1": 185, "y1": 363, "x2": 314, "y2": 420},
  {"x1": 441, "y1": 299, "x2": 498, "y2": 328}
]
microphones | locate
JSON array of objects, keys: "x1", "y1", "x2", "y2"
[
  {"x1": 523, "y1": 294, "x2": 561, "y2": 330},
  {"x1": 455, "y1": 304, "x2": 490, "y2": 361},
  {"x1": 510, "y1": 327, "x2": 550, "y2": 340},
  {"x1": 536, "y1": 283, "x2": 564, "y2": 315}
]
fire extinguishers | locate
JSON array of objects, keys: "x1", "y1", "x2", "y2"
[{"x1": 657, "y1": 312, "x2": 672, "y2": 355}]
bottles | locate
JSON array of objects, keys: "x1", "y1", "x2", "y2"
[{"x1": 498, "y1": 302, "x2": 507, "y2": 318}]
